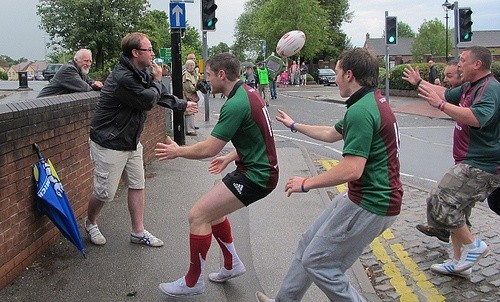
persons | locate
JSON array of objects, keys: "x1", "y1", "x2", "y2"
[
  {"x1": 402, "y1": 46, "x2": 500, "y2": 277},
  {"x1": 290, "y1": 60, "x2": 300, "y2": 85},
  {"x1": 245, "y1": 62, "x2": 277, "y2": 106},
  {"x1": 255, "y1": 47, "x2": 404, "y2": 302},
  {"x1": 301, "y1": 62, "x2": 308, "y2": 86},
  {"x1": 429, "y1": 60, "x2": 438, "y2": 84},
  {"x1": 37, "y1": 49, "x2": 103, "y2": 98},
  {"x1": 84, "y1": 32, "x2": 198, "y2": 247},
  {"x1": 182, "y1": 54, "x2": 200, "y2": 136},
  {"x1": 282, "y1": 70, "x2": 289, "y2": 87},
  {"x1": 155, "y1": 53, "x2": 279, "y2": 297}
]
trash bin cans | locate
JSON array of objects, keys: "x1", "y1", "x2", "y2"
[{"x1": 17, "y1": 71, "x2": 28, "y2": 88}]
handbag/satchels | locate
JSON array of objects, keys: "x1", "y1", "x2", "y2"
[{"x1": 196, "y1": 79, "x2": 210, "y2": 94}]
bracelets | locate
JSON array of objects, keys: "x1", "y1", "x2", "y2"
[
  {"x1": 301, "y1": 178, "x2": 310, "y2": 192},
  {"x1": 291, "y1": 122, "x2": 297, "y2": 133},
  {"x1": 438, "y1": 100, "x2": 445, "y2": 111},
  {"x1": 417, "y1": 78, "x2": 423, "y2": 85}
]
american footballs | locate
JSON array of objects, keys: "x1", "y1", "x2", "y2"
[{"x1": 276, "y1": 30, "x2": 306, "y2": 58}]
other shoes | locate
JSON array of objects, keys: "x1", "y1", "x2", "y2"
[
  {"x1": 416, "y1": 223, "x2": 451, "y2": 243},
  {"x1": 187, "y1": 132, "x2": 198, "y2": 136},
  {"x1": 254, "y1": 290, "x2": 276, "y2": 302},
  {"x1": 209, "y1": 264, "x2": 247, "y2": 283},
  {"x1": 158, "y1": 276, "x2": 205, "y2": 297}
]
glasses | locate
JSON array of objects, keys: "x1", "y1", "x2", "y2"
[{"x1": 136, "y1": 46, "x2": 154, "y2": 53}]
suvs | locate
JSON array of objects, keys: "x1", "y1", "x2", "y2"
[{"x1": 41, "y1": 64, "x2": 65, "y2": 82}]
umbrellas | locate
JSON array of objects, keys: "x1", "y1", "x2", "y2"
[{"x1": 32, "y1": 143, "x2": 86, "y2": 259}]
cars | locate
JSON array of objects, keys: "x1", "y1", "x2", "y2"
[
  {"x1": 27, "y1": 71, "x2": 45, "y2": 81},
  {"x1": 314, "y1": 68, "x2": 338, "y2": 86}
]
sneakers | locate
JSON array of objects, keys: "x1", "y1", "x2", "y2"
[
  {"x1": 130, "y1": 228, "x2": 164, "y2": 246},
  {"x1": 431, "y1": 258, "x2": 473, "y2": 279},
  {"x1": 85, "y1": 216, "x2": 106, "y2": 246},
  {"x1": 454, "y1": 239, "x2": 489, "y2": 272}
]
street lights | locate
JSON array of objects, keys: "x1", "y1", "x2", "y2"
[{"x1": 441, "y1": 0, "x2": 453, "y2": 63}]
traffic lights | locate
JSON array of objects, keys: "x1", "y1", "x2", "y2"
[
  {"x1": 457, "y1": 7, "x2": 475, "y2": 44},
  {"x1": 199, "y1": 0, "x2": 218, "y2": 32},
  {"x1": 384, "y1": 16, "x2": 398, "y2": 45}
]
